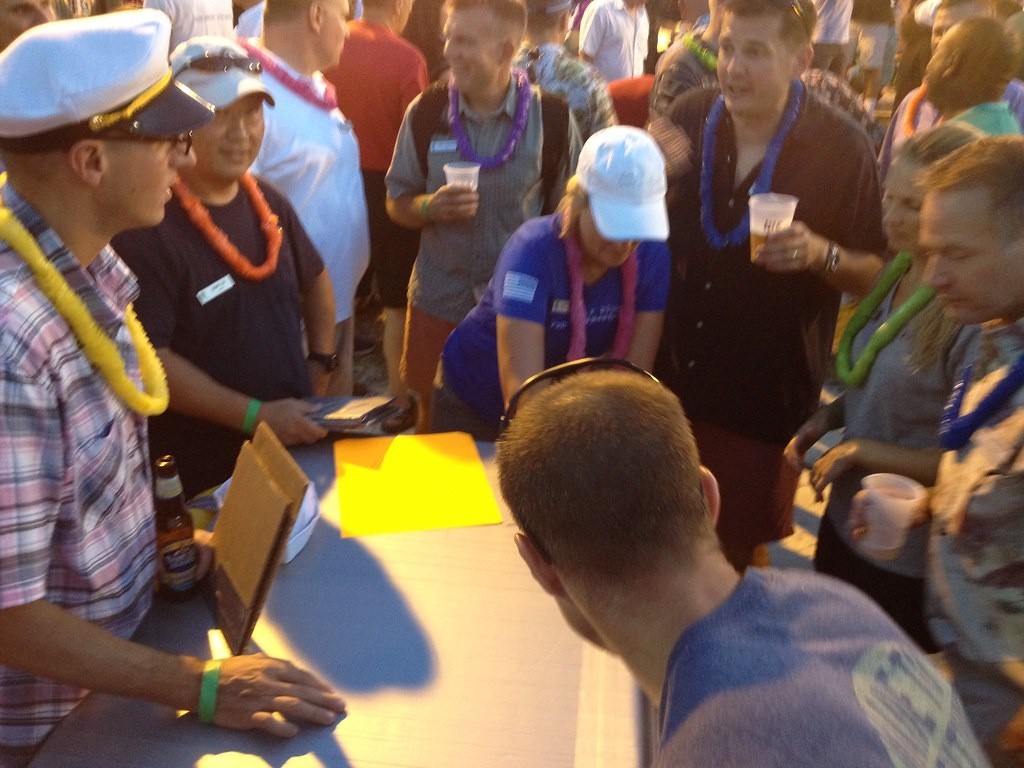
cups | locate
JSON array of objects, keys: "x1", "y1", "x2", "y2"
[
  {"x1": 748, "y1": 190, "x2": 800, "y2": 263},
  {"x1": 443, "y1": 160, "x2": 481, "y2": 192},
  {"x1": 861, "y1": 473, "x2": 927, "y2": 563}
]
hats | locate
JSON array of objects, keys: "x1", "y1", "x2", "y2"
[
  {"x1": 0, "y1": 11, "x2": 217, "y2": 154},
  {"x1": 576, "y1": 124, "x2": 671, "y2": 242},
  {"x1": 168, "y1": 34, "x2": 276, "y2": 113}
]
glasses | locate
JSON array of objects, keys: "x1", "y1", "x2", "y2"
[
  {"x1": 59, "y1": 132, "x2": 195, "y2": 159},
  {"x1": 502, "y1": 353, "x2": 660, "y2": 565},
  {"x1": 170, "y1": 54, "x2": 262, "y2": 85}
]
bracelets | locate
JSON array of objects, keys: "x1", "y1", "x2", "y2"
[
  {"x1": 241, "y1": 398, "x2": 262, "y2": 434},
  {"x1": 422, "y1": 195, "x2": 430, "y2": 223},
  {"x1": 824, "y1": 241, "x2": 840, "y2": 278},
  {"x1": 198, "y1": 660, "x2": 222, "y2": 724}
]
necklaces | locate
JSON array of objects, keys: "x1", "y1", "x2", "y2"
[
  {"x1": 0, "y1": 204, "x2": 169, "y2": 416},
  {"x1": 699, "y1": 80, "x2": 803, "y2": 251},
  {"x1": 937, "y1": 352, "x2": 1024, "y2": 454},
  {"x1": 904, "y1": 83, "x2": 944, "y2": 136},
  {"x1": 565, "y1": 235, "x2": 638, "y2": 361},
  {"x1": 237, "y1": 35, "x2": 337, "y2": 111},
  {"x1": 172, "y1": 171, "x2": 283, "y2": 281},
  {"x1": 683, "y1": 32, "x2": 718, "y2": 72},
  {"x1": 447, "y1": 67, "x2": 532, "y2": 169},
  {"x1": 836, "y1": 251, "x2": 935, "y2": 387}
]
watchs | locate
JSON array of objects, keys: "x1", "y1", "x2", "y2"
[{"x1": 308, "y1": 351, "x2": 339, "y2": 373}]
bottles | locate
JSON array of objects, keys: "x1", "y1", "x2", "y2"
[{"x1": 150, "y1": 454, "x2": 201, "y2": 605}]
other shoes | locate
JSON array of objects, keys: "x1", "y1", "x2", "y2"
[
  {"x1": 353, "y1": 337, "x2": 377, "y2": 356},
  {"x1": 356, "y1": 277, "x2": 378, "y2": 310},
  {"x1": 383, "y1": 400, "x2": 418, "y2": 433}
]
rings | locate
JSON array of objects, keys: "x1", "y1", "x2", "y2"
[{"x1": 792, "y1": 249, "x2": 798, "y2": 258}]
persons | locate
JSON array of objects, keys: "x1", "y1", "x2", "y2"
[
  {"x1": 0, "y1": 0, "x2": 1024, "y2": 768},
  {"x1": 495, "y1": 356, "x2": 995, "y2": 768}
]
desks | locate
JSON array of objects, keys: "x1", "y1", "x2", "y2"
[{"x1": 27, "y1": 437, "x2": 652, "y2": 768}]
